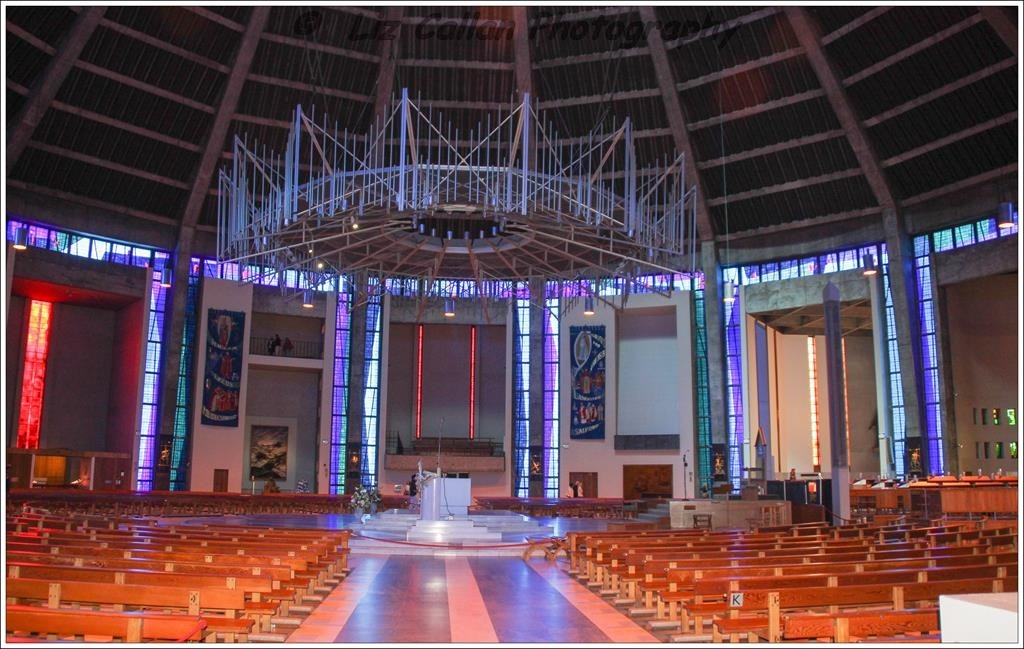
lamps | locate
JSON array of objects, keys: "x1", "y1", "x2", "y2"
[
  {"x1": 997, "y1": 200, "x2": 1014, "y2": 229},
  {"x1": 13, "y1": 226, "x2": 28, "y2": 250},
  {"x1": 861, "y1": 254, "x2": 878, "y2": 275},
  {"x1": 723, "y1": 282, "x2": 735, "y2": 303},
  {"x1": 443, "y1": 297, "x2": 456, "y2": 317},
  {"x1": 302, "y1": 290, "x2": 316, "y2": 309},
  {"x1": 583, "y1": 296, "x2": 595, "y2": 315},
  {"x1": 159, "y1": 268, "x2": 172, "y2": 289}
]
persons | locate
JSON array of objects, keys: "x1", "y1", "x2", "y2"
[
  {"x1": 352, "y1": 484, "x2": 382, "y2": 514},
  {"x1": 267, "y1": 334, "x2": 280, "y2": 355},
  {"x1": 568, "y1": 480, "x2": 583, "y2": 497},
  {"x1": 284, "y1": 336, "x2": 293, "y2": 355},
  {"x1": 409, "y1": 474, "x2": 418, "y2": 510},
  {"x1": 296, "y1": 479, "x2": 309, "y2": 493}
]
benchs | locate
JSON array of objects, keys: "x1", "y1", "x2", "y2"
[
  {"x1": 563, "y1": 516, "x2": 1017, "y2": 643},
  {"x1": 6, "y1": 511, "x2": 353, "y2": 643}
]
